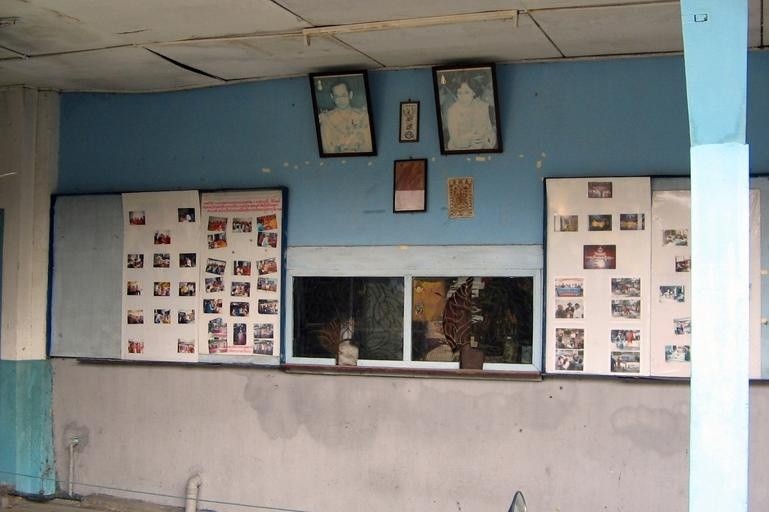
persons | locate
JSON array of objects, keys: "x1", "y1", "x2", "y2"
[
  {"x1": 556, "y1": 182, "x2": 691, "y2": 372},
  {"x1": 127, "y1": 210, "x2": 278, "y2": 356},
  {"x1": 319, "y1": 79, "x2": 372, "y2": 155},
  {"x1": 447, "y1": 74, "x2": 499, "y2": 152}
]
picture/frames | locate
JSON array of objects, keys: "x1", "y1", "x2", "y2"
[
  {"x1": 399, "y1": 101, "x2": 420, "y2": 143},
  {"x1": 432, "y1": 61, "x2": 503, "y2": 155},
  {"x1": 393, "y1": 158, "x2": 427, "y2": 213},
  {"x1": 308, "y1": 70, "x2": 378, "y2": 158}
]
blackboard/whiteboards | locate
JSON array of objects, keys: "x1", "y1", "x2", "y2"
[
  {"x1": 540, "y1": 174, "x2": 769, "y2": 384},
  {"x1": 46, "y1": 186, "x2": 288, "y2": 371}
]
